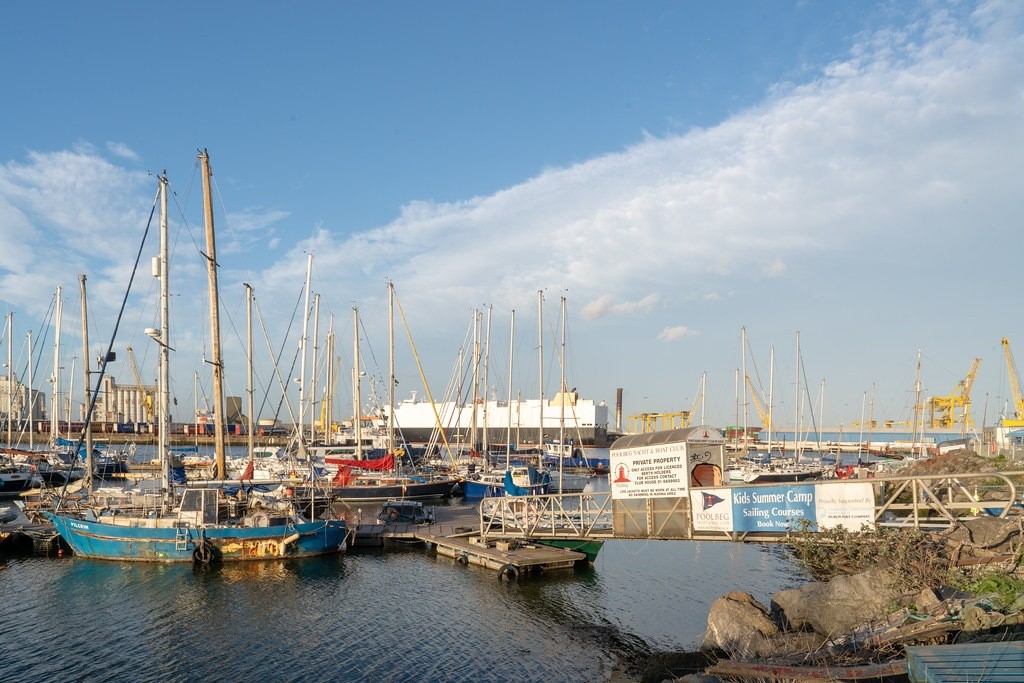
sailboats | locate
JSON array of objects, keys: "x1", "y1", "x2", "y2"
[
  {"x1": 722, "y1": 323, "x2": 887, "y2": 484},
  {"x1": 0, "y1": 143, "x2": 613, "y2": 565}
]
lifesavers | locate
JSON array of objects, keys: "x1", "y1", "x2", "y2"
[
  {"x1": 455, "y1": 527, "x2": 472, "y2": 534},
  {"x1": 497, "y1": 564, "x2": 520, "y2": 582},
  {"x1": 192, "y1": 544, "x2": 212, "y2": 564},
  {"x1": 456, "y1": 555, "x2": 469, "y2": 565}
]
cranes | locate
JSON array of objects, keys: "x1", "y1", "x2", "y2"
[
  {"x1": 998, "y1": 336, "x2": 1024, "y2": 429},
  {"x1": 921, "y1": 358, "x2": 984, "y2": 430}
]
